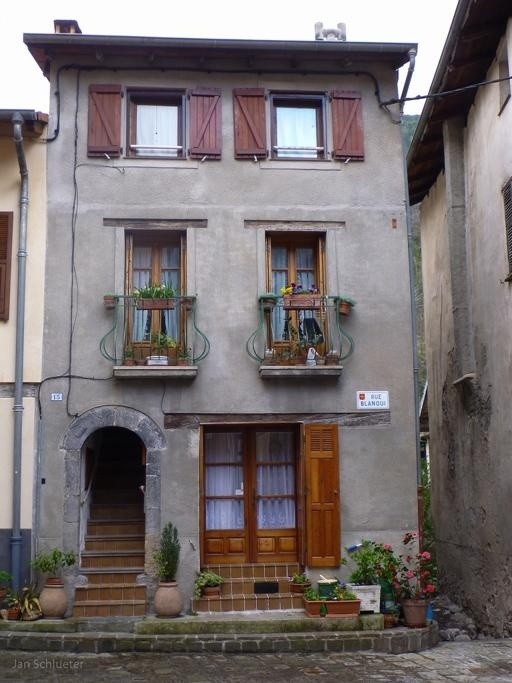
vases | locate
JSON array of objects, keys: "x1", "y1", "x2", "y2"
[
  {"x1": 400, "y1": 599, "x2": 427, "y2": 628},
  {"x1": 283, "y1": 294, "x2": 322, "y2": 309}
]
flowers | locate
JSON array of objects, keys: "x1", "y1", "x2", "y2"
[
  {"x1": 382, "y1": 532, "x2": 439, "y2": 599},
  {"x1": 280, "y1": 282, "x2": 318, "y2": 297}
]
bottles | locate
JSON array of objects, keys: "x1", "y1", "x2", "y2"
[{"x1": 320, "y1": 602, "x2": 326, "y2": 617}]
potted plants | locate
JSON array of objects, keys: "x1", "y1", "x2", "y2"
[
  {"x1": 344, "y1": 568, "x2": 381, "y2": 614},
  {"x1": 259, "y1": 292, "x2": 277, "y2": 313},
  {"x1": 8, "y1": 598, "x2": 20, "y2": 619},
  {"x1": 300, "y1": 587, "x2": 362, "y2": 617},
  {"x1": 182, "y1": 295, "x2": 195, "y2": 311},
  {"x1": 193, "y1": 567, "x2": 225, "y2": 601},
  {"x1": 133, "y1": 281, "x2": 176, "y2": 310},
  {"x1": 335, "y1": 295, "x2": 356, "y2": 316},
  {"x1": 289, "y1": 572, "x2": 311, "y2": 593},
  {"x1": 151, "y1": 521, "x2": 184, "y2": 619},
  {"x1": 103, "y1": 291, "x2": 116, "y2": 310},
  {"x1": 0, "y1": 570, "x2": 14, "y2": 601},
  {"x1": 29, "y1": 546, "x2": 76, "y2": 620},
  {"x1": 124, "y1": 329, "x2": 193, "y2": 365},
  {"x1": 342, "y1": 538, "x2": 398, "y2": 628}
]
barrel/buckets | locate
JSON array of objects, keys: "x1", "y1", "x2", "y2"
[{"x1": 317, "y1": 579, "x2": 338, "y2": 600}]
